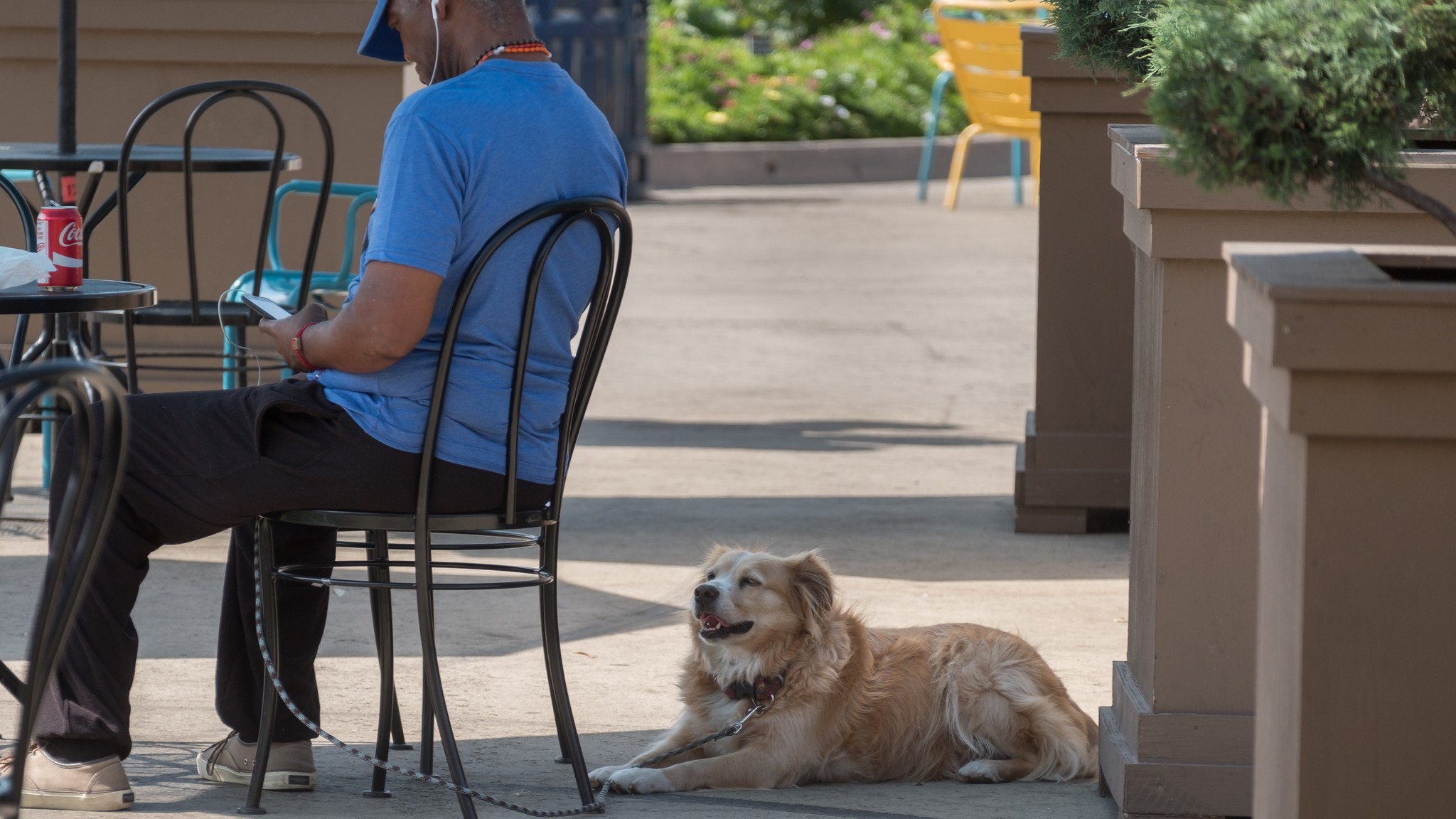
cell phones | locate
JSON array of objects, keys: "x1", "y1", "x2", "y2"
[{"x1": 241, "y1": 294, "x2": 292, "y2": 320}]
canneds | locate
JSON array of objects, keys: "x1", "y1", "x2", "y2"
[{"x1": 36, "y1": 201, "x2": 83, "y2": 292}]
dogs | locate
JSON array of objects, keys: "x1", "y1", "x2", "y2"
[{"x1": 583, "y1": 534, "x2": 1100, "y2": 799}]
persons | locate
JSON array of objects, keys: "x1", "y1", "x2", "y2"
[{"x1": 1, "y1": 0, "x2": 630, "y2": 807}]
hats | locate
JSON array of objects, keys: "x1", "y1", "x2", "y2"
[{"x1": 356, "y1": 0, "x2": 406, "y2": 61}]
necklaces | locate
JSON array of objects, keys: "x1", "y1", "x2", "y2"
[{"x1": 474, "y1": 38, "x2": 549, "y2": 63}]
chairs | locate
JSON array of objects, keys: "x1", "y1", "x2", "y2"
[
  {"x1": 0, "y1": 76, "x2": 635, "y2": 819},
  {"x1": 912, "y1": 0, "x2": 1063, "y2": 214}
]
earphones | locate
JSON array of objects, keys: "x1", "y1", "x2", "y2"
[{"x1": 431, "y1": 0, "x2": 439, "y2": 19}]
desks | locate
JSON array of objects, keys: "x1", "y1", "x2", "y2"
[
  {"x1": 0, "y1": 275, "x2": 159, "y2": 453},
  {"x1": 0, "y1": 137, "x2": 305, "y2": 504}
]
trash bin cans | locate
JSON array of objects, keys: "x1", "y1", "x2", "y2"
[{"x1": 524, "y1": 0, "x2": 651, "y2": 202}]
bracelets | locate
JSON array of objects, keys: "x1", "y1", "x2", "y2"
[{"x1": 289, "y1": 322, "x2": 315, "y2": 373}]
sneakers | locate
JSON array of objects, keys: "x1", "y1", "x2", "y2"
[
  {"x1": 0, "y1": 740, "x2": 134, "y2": 809},
  {"x1": 196, "y1": 729, "x2": 317, "y2": 789}
]
